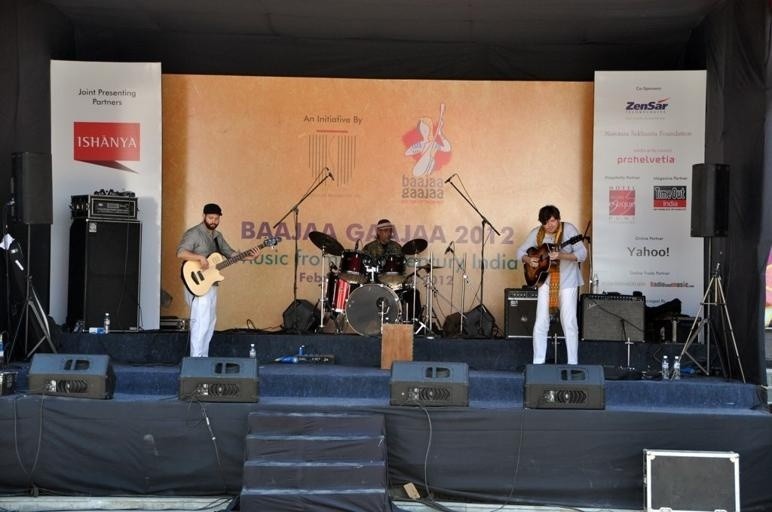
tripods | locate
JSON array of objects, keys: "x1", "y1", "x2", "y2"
[
  {"x1": 6, "y1": 223, "x2": 59, "y2": 366},
  {"x1": 410, "y1": 253, "x2": 468, "y2": 339},
  {"x1": 304, "y1": 246, "x2": 342, "y2": 334},
  {"x1": 670, "y1": 263, "x2": 747, "y2": 384}
]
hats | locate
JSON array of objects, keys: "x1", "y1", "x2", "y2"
[
  {"x1": 204, "y1": 204, "x2": 222, "y2": 215},
  {"x1": 376, "y1": 219, "x2": 392, "y2": 227}
]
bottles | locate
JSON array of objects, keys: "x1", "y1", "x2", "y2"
[
  {"x1": 673, "y1": 356, "x2": 681, "y2": 380},
  {"x1": 104, "y1": 313, "x2": 111, "y2": 334},
  {"x1": 661, "y1": 356, "x2": 670, "y2": 381},
  {"x1": 249, "y1": 344, "x2": 257, "y2": 358}
]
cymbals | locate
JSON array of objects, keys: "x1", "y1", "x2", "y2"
[
  {"x1": 416, "y1": 264, "x2": 443, "y2": 270},
  {"x1": 402, "y1": 239, "x2": 427, "y2": 255},
  {"x1": 309, "y1": 231, "x2": 344, "y2": 256}
]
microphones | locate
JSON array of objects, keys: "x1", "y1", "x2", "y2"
[
  {"x1": 444, "y1": 173, "x2": 456, "y2": 183},
  {"x1": 325, "y1": 167, "x2": 334, "y2": 181},
  {"x1": 444, "y1": 240, "x2": 453, "y2": 254}
]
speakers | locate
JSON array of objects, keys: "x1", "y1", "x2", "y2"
[
  {"x1": 65, "y1": 219, "x2": 142, "y2": 334},
  {"x1": 691, "y1": 163, "x2": 730, "y2": 237},
  {"x1": 523, "y1": 364, "x2": 605, "y2": 410},
  {"x1": 178, "y1": 357, "x2": 261, "y2": 403},
  {"x1": 641, "y1": 448, "x2": 742, "y2": 511},
  {"x1": 389, "y1": 359, "x2": 470, "y2": 407},
  {"x1": 580, "y1": 293, "x2": 646, "y2": 344},
  {"x1": 9, "y1": 151, "x2": 54, "y2": 224},
  {"x1": 503, "y1": 288, "x2": 538, "y2": 338},
  {"x1": 26, "y1": 352, "x2": 116, "y2": 400},
  {"x1": 283, "y1": 300, "x2": 321, "y2": 334},
  {"x1": 463, "y1": 305, "x2": 495, "y2": 338}
]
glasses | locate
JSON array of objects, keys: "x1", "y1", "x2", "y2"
[{"x1": 377, "y1": 228, "x2": 392, "y2": 232}]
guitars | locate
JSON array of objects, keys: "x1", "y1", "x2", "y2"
[
  {"x1": 182, "y1": 236, "x2": 282, "y2": 297},
  {"x1": 523, "y1": 234, "x2": 584, "y2": 290}
]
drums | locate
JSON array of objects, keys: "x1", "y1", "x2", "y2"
[
  {"x1": 378, "y1": 253, "x2": 406, "y2": 283},
  {"x1": 324, "y1": 278, "x2": 358, "y2": 315},
  {"x1": 345, "y1": 283, "x2": 402, "y2": 336},
  {"x1": 338, "y1": 249, "x2": 373, "y2": 285},
  {"x1": 392, "y1": 284, "x2": 421, "y2": 322}
]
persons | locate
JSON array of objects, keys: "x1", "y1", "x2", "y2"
[
  {"x1": 404, "y1": 116, "x2": 452, "y2": 176},
  {"x1": 360, "y1": 217, "x2": 422, "y2": 320},
  {"x1": 176, "y1": 203, "x2": 263, "y2": 357},
  {"x1": 515, "y1": 203, "x2": 588, "y2": 366}
]
